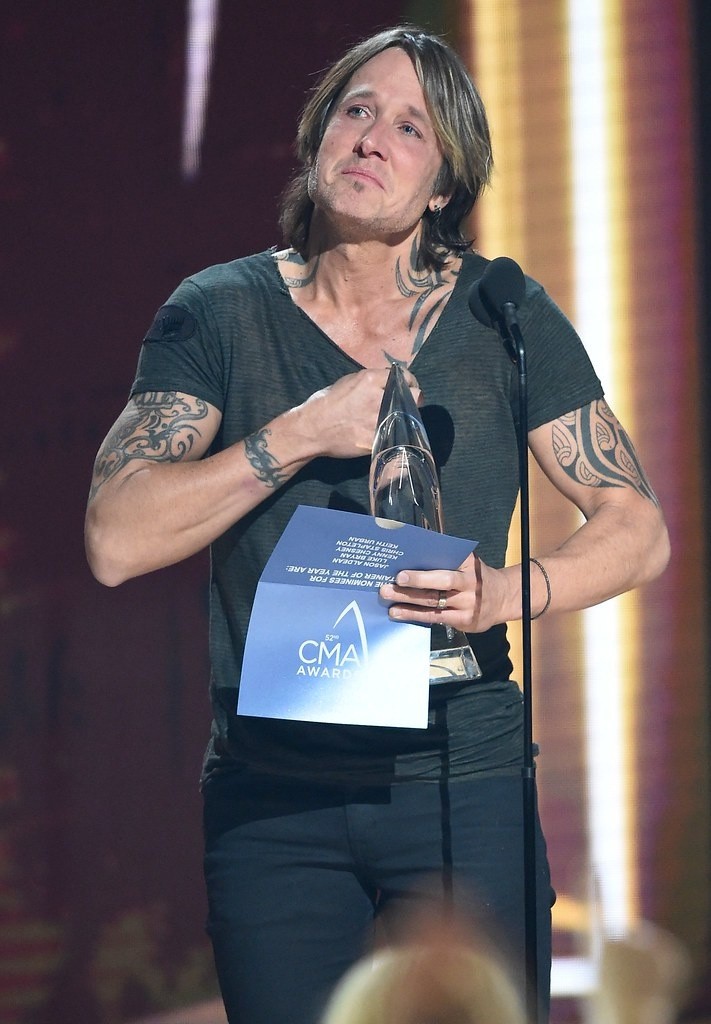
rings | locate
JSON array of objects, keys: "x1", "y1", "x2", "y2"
[{"x1": 437, "y1": 591, "x2": 446, "y2": 610}]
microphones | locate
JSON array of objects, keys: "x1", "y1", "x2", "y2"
[{"x1": 467, "y1": 257, "x2": 526, "y2": 364}]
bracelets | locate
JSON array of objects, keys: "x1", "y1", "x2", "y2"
[{"x1": 530, "y1": 557, "x2": 552, "y2": 620}]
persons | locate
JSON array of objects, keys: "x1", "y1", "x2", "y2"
[
  {"x1": 83, "y1": 26, "x2": 672, "y2": 1024},
  {"x1": 317, "y1": 940, "x2": 529, "y2": 1024}
]
